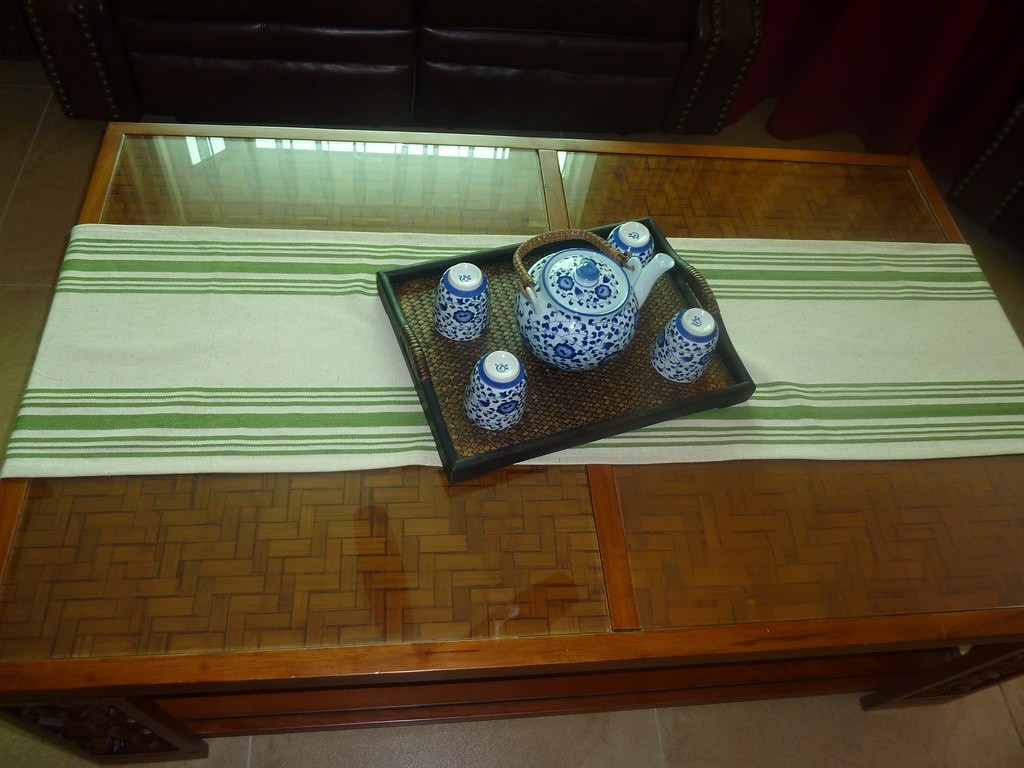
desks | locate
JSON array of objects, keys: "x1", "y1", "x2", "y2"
[{"x1": 0, "y1": 118, "x2": 1024, "y2": 768}]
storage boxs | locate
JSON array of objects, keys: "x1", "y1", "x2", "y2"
[{"x1": 375, "y1": 218, "x2": 758, "y2": 487}]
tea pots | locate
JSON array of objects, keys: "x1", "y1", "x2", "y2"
[{"x1": 509, "y1": 227, "x2": 677, "y2": 373}]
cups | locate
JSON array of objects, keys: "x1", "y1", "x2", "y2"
[
  {"x1": 605, "y1": 221, "x2": 655, "y2": 270},
  {"x1": 432, "y1": 262, "x2": 490, "y2": 341},
  {"x1": 464, "y1": 350, "x2": 527, "y2": 431},
  {"x1": 649, "y1": 307, "x2": 720, "y2": 384}
]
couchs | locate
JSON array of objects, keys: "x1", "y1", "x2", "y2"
[{"x1": 19, "y1": 0, "x2": 763, "y2": 138}]
